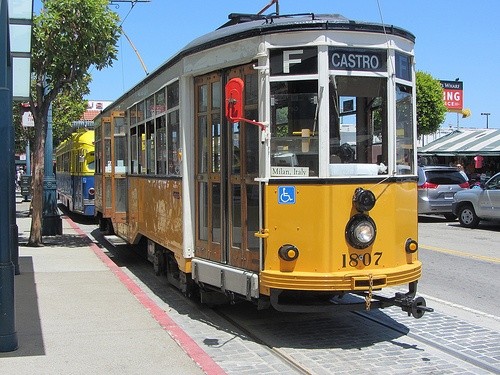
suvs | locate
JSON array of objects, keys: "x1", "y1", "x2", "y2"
[{"x1": 397, "y1": 164, "x2": 471, "y2": 221}]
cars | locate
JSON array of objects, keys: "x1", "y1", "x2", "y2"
[{"x1": 452, "y1": 172, "x2": 500, "y2": 229}]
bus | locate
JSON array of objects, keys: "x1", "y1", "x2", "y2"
[
  {"x1": 56, "y1": 130, "x2": 155, "y2": 217},
  {"x1": 93, "y1": 13, "x2": 434, "y2": 319}
]
